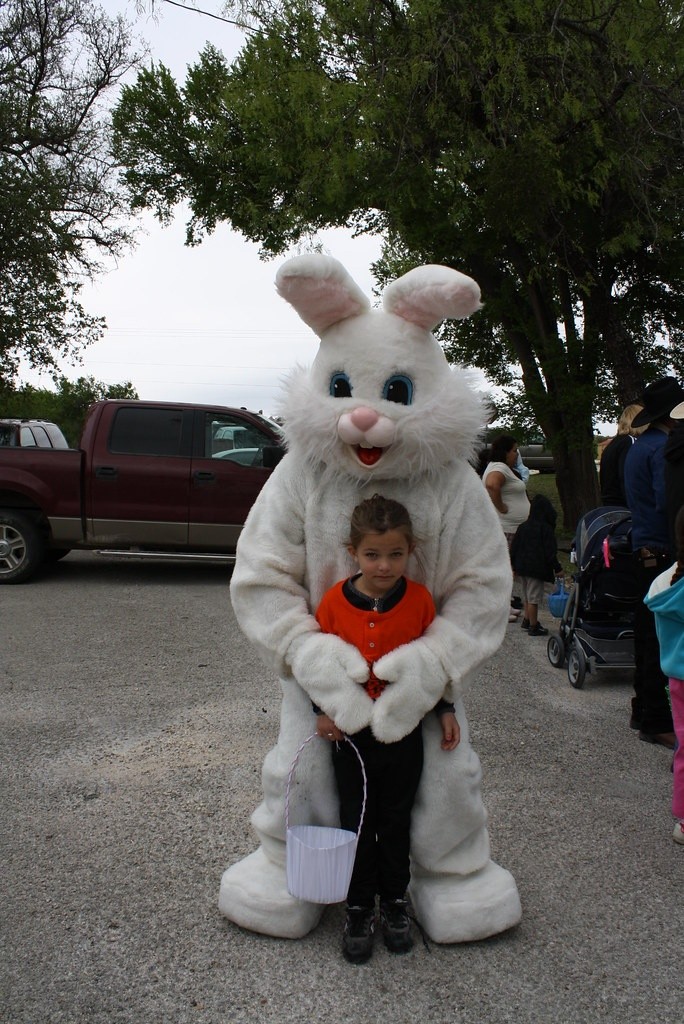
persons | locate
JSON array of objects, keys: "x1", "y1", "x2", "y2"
[
  {"x1": 481, "y1": 435, "x2": 565, "y2": 635},
  {"x1": 313, "y1": 494, "x2": 461, "y2": 965},
  {"x1": 599, "y1": 376, "x2": 684, "y2": 847}
]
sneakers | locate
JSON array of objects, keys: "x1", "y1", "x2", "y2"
[
  {"x1": 341, "y1": 906, "x2": 375, "y2": 964},
  {"x1": 379, "y1": 896, "x2": 431, "y2": 954}
]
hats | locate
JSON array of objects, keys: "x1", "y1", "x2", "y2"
[
  {"x1": 631, "y1": 376, "x2": 684, "y2": 428},
  {"x1": 670, "y1": 401, "x2": 684, "y2": 419}
]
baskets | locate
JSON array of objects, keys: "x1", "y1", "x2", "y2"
[
  {"x1": 284, "y1": 731, "x2": 366, "y2": 904},
  {"x1": 548, "y1": 578, "x2": 569, "y2": 617}
]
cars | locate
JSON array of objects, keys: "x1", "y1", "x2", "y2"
[
  {"x1": 212, "y1": 448, "x2": 261, "y2": 464},
  {"x1": 478, "y1": 426, "x2": 556, "y2": 474}
]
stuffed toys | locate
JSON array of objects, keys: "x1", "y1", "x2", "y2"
[{"x1": 216, "y1": 251, "x2": 522, "y2": 948}]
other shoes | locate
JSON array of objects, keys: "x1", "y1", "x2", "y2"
[
  {"x1": 510, "y1": 606, "x2": 521, "y2": 615},
  {"x1": 672, "y1": 819, "x2": 684, "y2": 844},
  {"x1": 521, "y1": 618, "x2": 530, "y2": 629},
  {"x1": 528, "y1": 621, "x2": 549, "y2": 636},
  {"x1": 508, "y1": 615, "x2": 518, "y2": 621},
  {"x1": 511, "y1": 596, "x2": 524, "y2": 609}
]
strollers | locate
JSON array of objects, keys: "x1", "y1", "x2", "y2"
[{"x1": 547, "y1": 506, "x2": 637, "y2": 688}]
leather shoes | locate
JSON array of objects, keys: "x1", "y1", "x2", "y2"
[
  {"x1": 631, "y1": 720, "x2": 641, "y2": 730},
  {"x1": 639, "y1": 731, "x2": 675, "y2": 749}
]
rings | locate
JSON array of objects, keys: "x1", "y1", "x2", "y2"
[{"x1": 328, "y1": 733, "x2": 333, "y2": 736}]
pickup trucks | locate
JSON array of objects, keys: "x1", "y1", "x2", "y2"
[{"x1": 0, "y1": 400, "x2": 289, "y2": 583}]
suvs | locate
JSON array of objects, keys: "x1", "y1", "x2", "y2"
[{"x1": 0, "y1": 417, "x2": 69, "y2": 449}]
